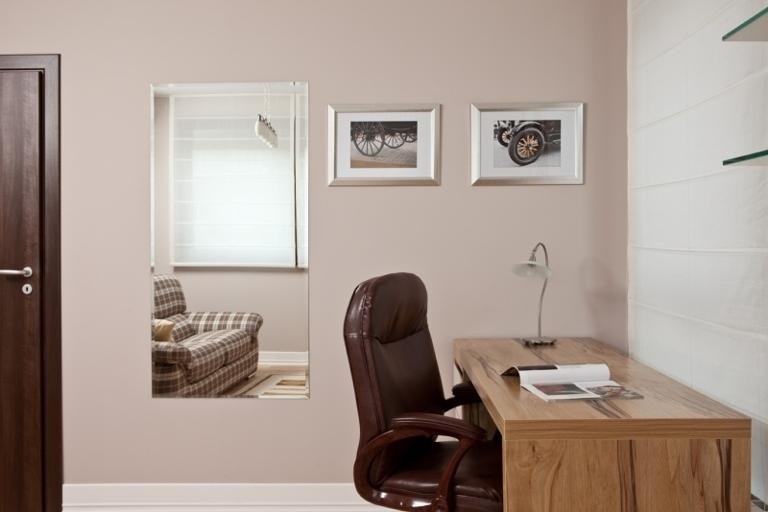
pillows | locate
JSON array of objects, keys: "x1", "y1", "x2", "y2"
[{"x1": 151, "y1": 318, "x2": 176, "y2": 342}]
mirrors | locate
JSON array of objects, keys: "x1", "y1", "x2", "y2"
[{"x1": 151, "y1": 82, "x2": 311, "y2": 402}]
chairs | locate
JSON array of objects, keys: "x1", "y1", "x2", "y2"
[{"x1": 343, "y1": 273, "x2": 503, "y2": 512}]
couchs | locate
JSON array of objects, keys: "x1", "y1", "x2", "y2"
[{"x1": 150, "y1": 273, "x2": 263, "y2": 402}]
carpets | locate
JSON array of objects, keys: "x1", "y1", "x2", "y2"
[{"x1": 219, "y1": 364, "x2": 311, "y2": 401}]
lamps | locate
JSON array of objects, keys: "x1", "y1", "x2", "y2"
[
  {"x1": 255, "y1": 81, "x2": 279, "y2": 150},
  {"x1": 514, "y1": 243, "x2": 557, "y2": 346}
]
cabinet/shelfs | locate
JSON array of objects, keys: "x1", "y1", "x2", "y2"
[{"x1": 722, "y1": 6, "x2": 768, "y2": 166}]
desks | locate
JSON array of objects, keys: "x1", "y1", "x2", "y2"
[{"x1": 451, "y1": 336, "x2": 754, "y2": 512}]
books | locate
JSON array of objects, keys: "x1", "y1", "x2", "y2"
[
  {"x1": 499, "y1": 363, "x2": 644, "y2": 401},
  {"x1": 261, "y1": 375, "x2": 308, "y2": 396}
]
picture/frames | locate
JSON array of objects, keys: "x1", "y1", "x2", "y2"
[
  {"x1": 329, "y1": 105, "x2": 441, "y2": 188},
  {"x1": 471, "y1": 101, "x2": 587, "y2": 186}
]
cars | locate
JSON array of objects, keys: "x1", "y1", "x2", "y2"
[{"x1": 494, "y1": 121, "x2": 561, "y2": 165}]
